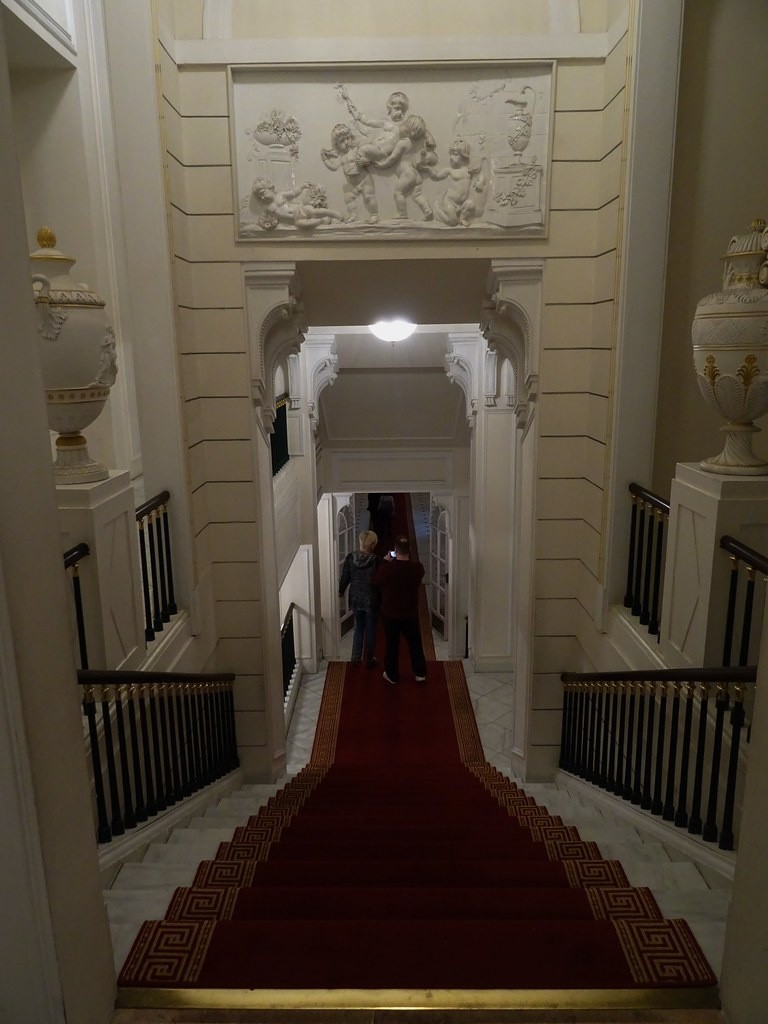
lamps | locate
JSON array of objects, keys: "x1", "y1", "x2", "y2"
[{"x1": 369, "y1": 324, "x2": 416, "y2": 343}]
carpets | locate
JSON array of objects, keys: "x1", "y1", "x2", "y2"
[{"x1": 111, "y1": 495, "x2": 718, "y2": 987}]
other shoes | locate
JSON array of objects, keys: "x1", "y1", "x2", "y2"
[{"x1": 368, "y1": 658, "x2": 378, "y2": 666}]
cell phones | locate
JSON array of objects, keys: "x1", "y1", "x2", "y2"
[{"x1": 390, "y1": 550, "x2": 396, "y2": 558}]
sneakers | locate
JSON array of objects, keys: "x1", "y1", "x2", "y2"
[
  {"x1": 415, "y1": 676, "x2": 425, "y2": 682},
  {"x1": 383, "y1": 671, "x2": 397, "y2": 685}
]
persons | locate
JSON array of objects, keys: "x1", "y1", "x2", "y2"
[
  {"x1": 366, "y1": 493, "x2": 381, "y2": 531},
  {"x1": 344, "y1": 91, "x2": 437, "y2": 177},
  {"x1": 251, "y1": 176, "x2": 344, "y2": 230},
  {"x1": 417, "y1": 137, "x2": 486, "y2": 228},
  {"x1": 373, "y1": 115, "x2": 434, "y2": 222},
  {"x1": 338, "y1": 530, "x2": 380, "y2": 667},
  {"x1": 320, "y1": 123, "x2": 380, "y2": 225},
  {"x1": 377, "y1": 493, "x2": 396, "y2": 534},
  {"x1": 373, "y1": 535, "x2": 428, "y2": 685}
]
